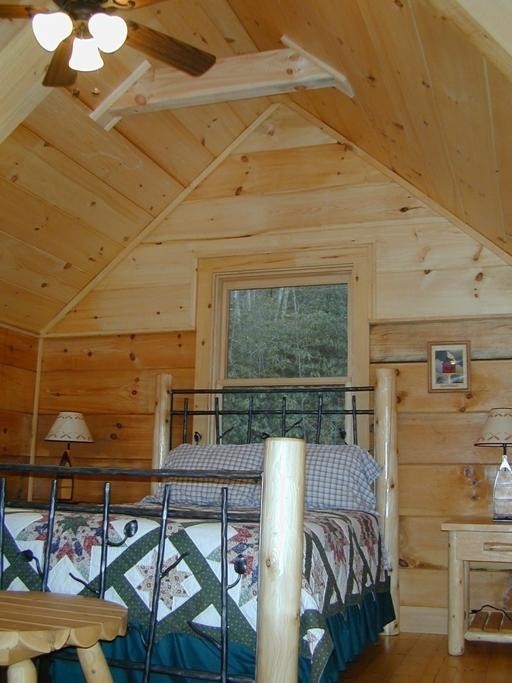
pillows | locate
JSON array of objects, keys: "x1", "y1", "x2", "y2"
[{"x1": 137, "y1": 442, "x2": 384, "y2": 518}]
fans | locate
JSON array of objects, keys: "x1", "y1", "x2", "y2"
[{"x1": 0, "y1": 0, "x2": 216, "y2": 88}]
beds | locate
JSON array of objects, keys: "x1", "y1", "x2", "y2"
[{"x1": 0, "y1": 366, "x2": 399, "y2": 683}]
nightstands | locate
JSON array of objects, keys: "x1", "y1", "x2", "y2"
[{"x1": 440, "y1": 516, "x2": 512, "y2": 656}]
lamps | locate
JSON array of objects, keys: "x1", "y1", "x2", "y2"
[
  {"x1": 32, "y1": 0, "x2": 128, "y2": 72},
  {"x1": 44, "y1": 411, "x2": 95, "y2": 503},
  {"x1": 474, "y1": 407, "x2": 512, "y2": 522}
]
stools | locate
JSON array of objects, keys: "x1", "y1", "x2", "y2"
[{"x1": 0, "y1": 591, "x2": 129, "y2": 683}]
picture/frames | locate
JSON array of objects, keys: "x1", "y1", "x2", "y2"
[{"x1": 427, "y1": 340, "x2": 471, "y2": 393}]
